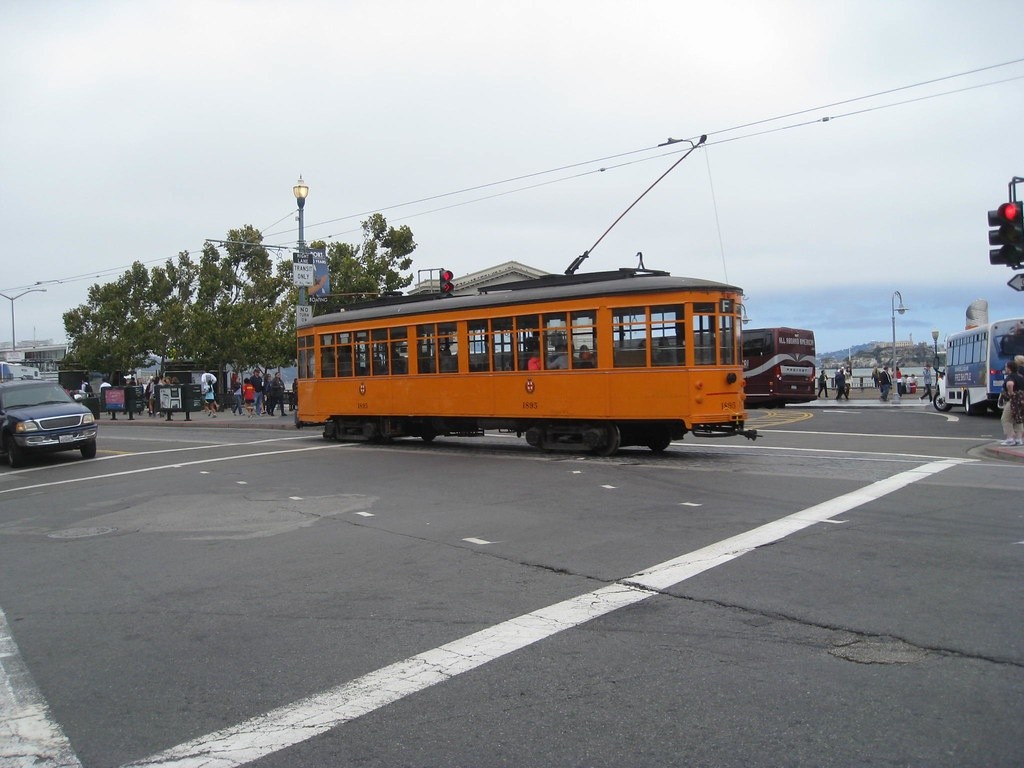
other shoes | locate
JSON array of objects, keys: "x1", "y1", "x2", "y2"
[
  {"x1": 1016, "y1": 439, "x2": 1022, "y2": 446},
  {"x1": 1001, "y1": 439, "x2": 1015, "y2": 445}
]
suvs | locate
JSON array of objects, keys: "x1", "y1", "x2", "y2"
[{"x1": 0, "y1": 377, "x2": 99, "y2": 468}]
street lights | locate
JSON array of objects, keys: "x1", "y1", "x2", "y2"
[
  {"x1": 932, "y1": 326, "x2": 940, "y2": 389},
  {"x1": 0, "y1": 289, "x2": 47, "y2": 349},
  {"x1": 292, "y1": 174, "x2": 311, "y2": 323},
  {"x1": 891, "y1": 290, "x2": 910, "y2": 404}
]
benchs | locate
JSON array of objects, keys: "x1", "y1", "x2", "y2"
[{"x1": 217, "y1": 393, "x2": 237, "y2": 411}]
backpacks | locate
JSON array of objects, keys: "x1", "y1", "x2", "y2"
[{"x1": 85, "y1": 383, "x2": 93, "y2": 392}]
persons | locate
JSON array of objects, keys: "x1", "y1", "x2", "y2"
[
  {"x1": 919, "y1": 362, "x2": 933, "y2": 403},
  {"x1": 129, "y1": 377, "x2": 137, "y2": 386},
  {"x1": 293, "y1": 378, "x2": 297, "y2": 392},
  {"x1": 579, "y1": 345, "x2": 594, "y2": 364},
  {"x1": 99, "y1": 377, "x2": 112, "y2": 391},
  {"x1": 201, "y1": 366, "x2": 220, "y2": 417},
  {"x1": 243, "y1": 368, "x2": 287, "y2": 418},
  {"x1": 80, "y1": 377, "x2": 94, "y2": 397},
  {"x1": 656, "y1": 337, "x2": 673, "y2": 363},
  {"x1": 120, "y1": 377, "x2": 127, "y2": 414},
  {"x1": 833, "y1": 366, "x2": 853, "y2": 400},
  {"x1": 546, "y1": 344, "x2": 575, "y2": 369},
  {"x1": 638, "y1": 339, "x2": 646, "y2": 348},
  {"x1": 891, "y1": 367, "x2": 919, "y2": 397},
  {"x1": 526, "y1": 336, "x2": 541, "y2": 370},
  {"x1": 998, "y1": 355, "x2": 1024, "y2": 446},
  {"x1": 145, "y1": 375, "x2": 179, "y2": 415},
  {"x1": 442, "y1": 343, "x2": 451, "y2": 355},
  {"x1": 230, "y1": 373, "x2": 245, "y2": 416},
  {"x1": 871, "y1": 367, "x2": 880, "y2": 388},
  {"x1": 818, "y1": 370, "x2": 828, "y2": 397},
  {"x1": 881, "y1": 367, "x2": 891, "y2": 401}
]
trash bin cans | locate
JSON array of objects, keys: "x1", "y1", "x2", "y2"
[
  {"x1": 101, "y1": 386, "x2": 144, "y2": 411},
  {"x1": 154, "y1": 384, "x2": 202, "y2": 412},
  {"x1": 81, "y1": 392, "x2": 101, "y2": 419}
]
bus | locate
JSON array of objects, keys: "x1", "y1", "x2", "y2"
[
  {"x1": 297, "y1": 266, "x2": 762, "y2": 460},
  {"x1": 615, "y1": 327, "x2": 816, "y2": 410},
  {"x1": 933, "y1": 318, "x2": 1024, "y2": 416}
]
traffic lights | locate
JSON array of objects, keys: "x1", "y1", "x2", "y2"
[
  {"x1": 439, "y1": 270, "x2": 455, "y2": 298},
  {"x1": 998, "y1": 202, "x2": 1024, "y2": 267},
  {"x1": 988, "y1": 210, "x2": 1008, "y2": 265}
]
